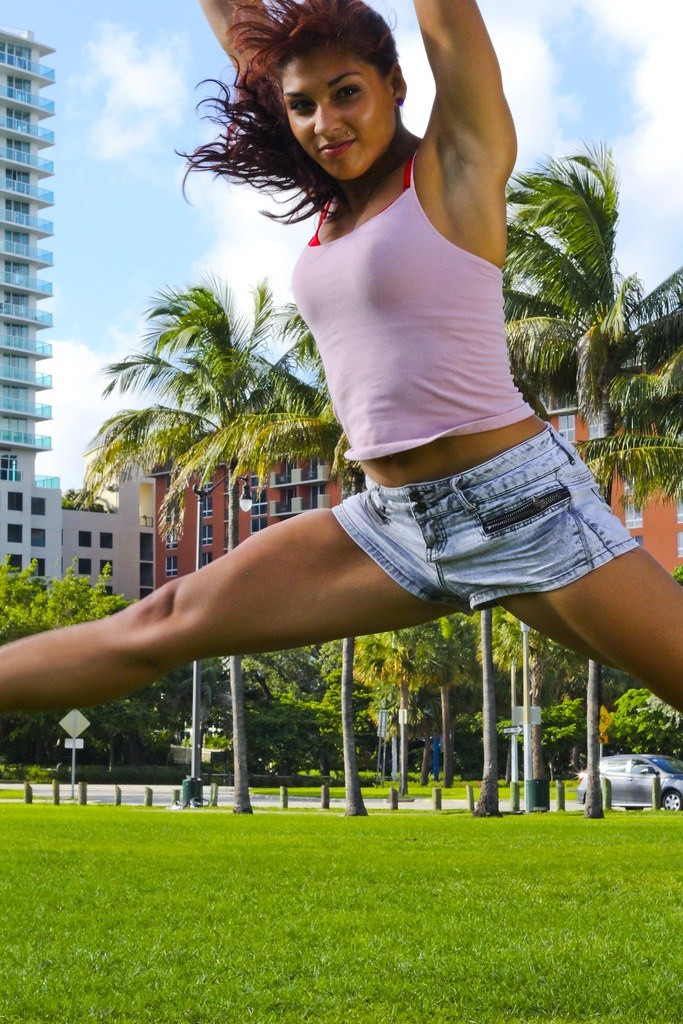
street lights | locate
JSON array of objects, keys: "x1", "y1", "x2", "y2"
[{"x1": 179, "y1": 474, "x2": 255, "y2": 804}]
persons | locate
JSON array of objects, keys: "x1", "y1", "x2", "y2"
[{"x1": 3, "y1": 0, "x2": 683, "y2": 718}]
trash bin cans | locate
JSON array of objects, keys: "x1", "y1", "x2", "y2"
[
  {"x1": 182, "y1": 778, "x2": 203, "y2": 807},
  {"x1": 526, "y1": 778, "x2": 550, "y2": 814}
]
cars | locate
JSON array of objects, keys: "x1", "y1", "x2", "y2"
[{"x1": 579, "y1": 754, "x2": 683, "y2": 811}]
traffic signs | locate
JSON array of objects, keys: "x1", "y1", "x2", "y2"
[{"x1": 503, "y1": 726, "x2": 523, "y2": 734}]
